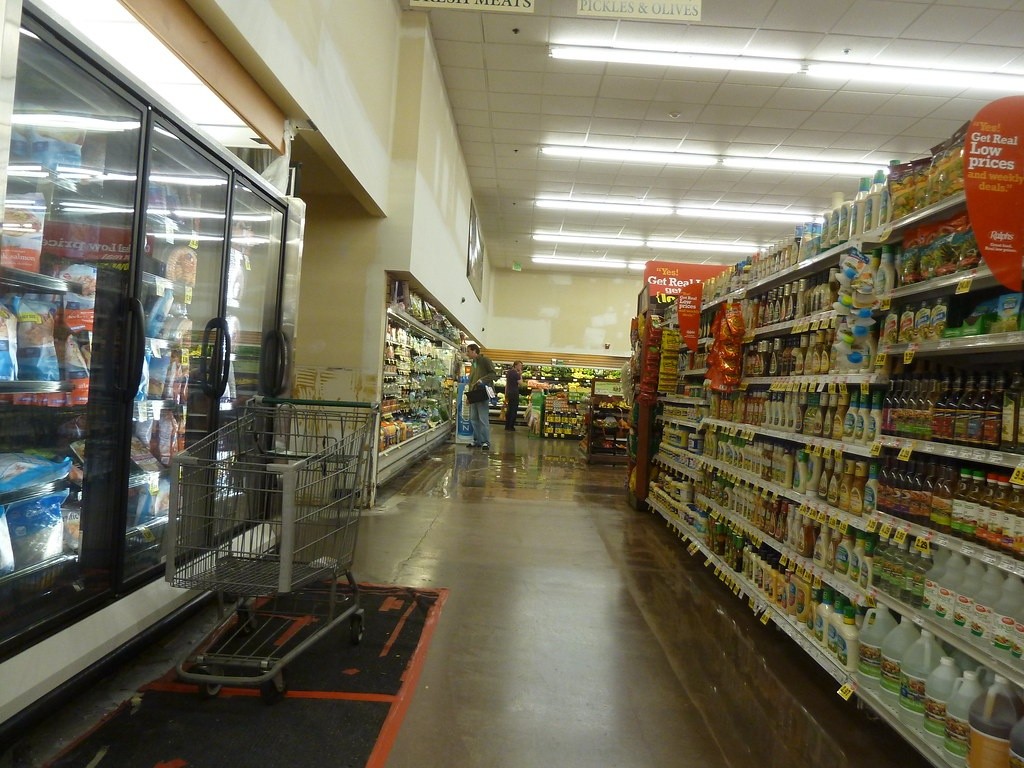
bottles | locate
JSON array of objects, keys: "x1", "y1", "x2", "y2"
[{"x1": 647, "y1": 159, "x2": 1024, "y2": 768}]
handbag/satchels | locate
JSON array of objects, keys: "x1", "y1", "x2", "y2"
[
  {"x1": 463, "y1": 382, "x2": 490, "y2": 404},
  {"x1": 519, "y1": 385, "x2": 532, "y2": 396}
]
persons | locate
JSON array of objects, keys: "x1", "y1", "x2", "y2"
[
  {"x1": 466, "y1": 344, "x2": 496, "y2": 450},
  {"x1": 505, "y1": 360, "x2": 523, "y2": 430}
]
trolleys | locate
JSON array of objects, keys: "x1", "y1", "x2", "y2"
[{"x1": 164, "y1": 393, "x2": 386, "y2": 704}]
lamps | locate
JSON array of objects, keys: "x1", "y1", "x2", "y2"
[{"x1": 377, "y1": 120, "x2": 1024, "y2": 768}]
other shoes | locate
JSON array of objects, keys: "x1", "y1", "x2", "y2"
[
  {"x1": 481, "y1": 443, "x2": 489, "y2": 450},
  {"x1": 504, "y1": 425, "x2": 515, "y2": 431},
  {"x1": 465, "y1": 440, "x2": 478, "y2": 448}
]
